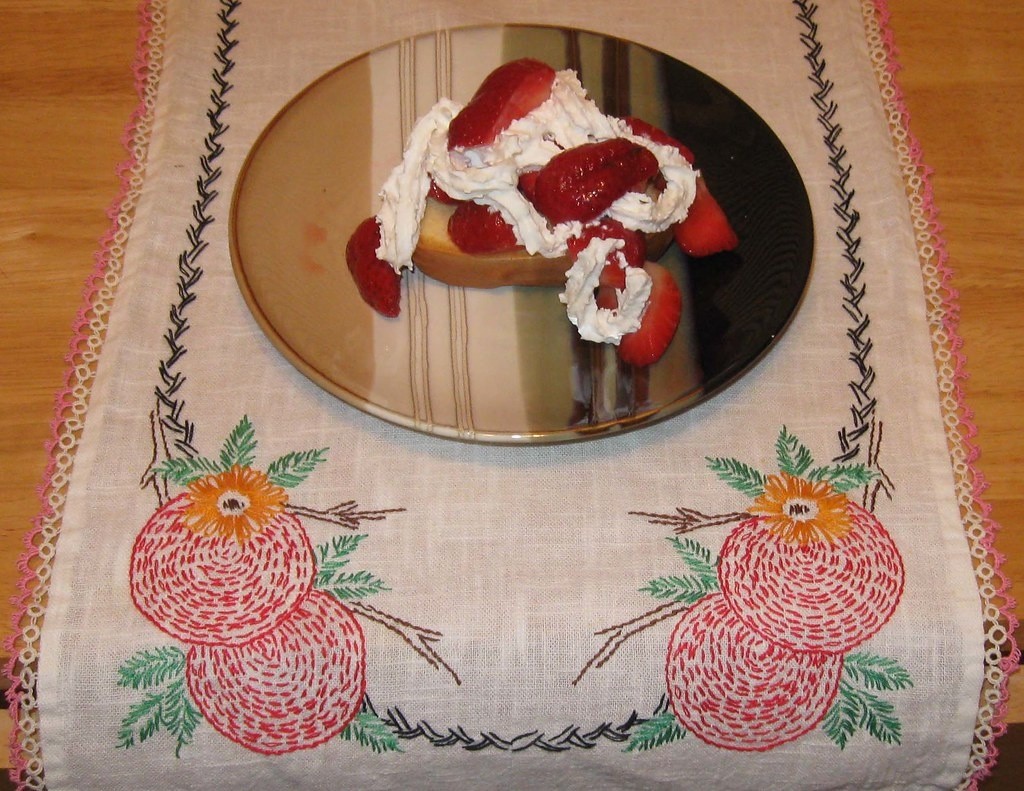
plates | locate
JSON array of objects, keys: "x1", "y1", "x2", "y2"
[{"x1": 228, "y1": 23, "x2": 817, "y2": 445}]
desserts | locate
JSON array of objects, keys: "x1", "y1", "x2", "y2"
[{"x1": 347, "y1": 56, "x2": 737, "y2": 366}]
